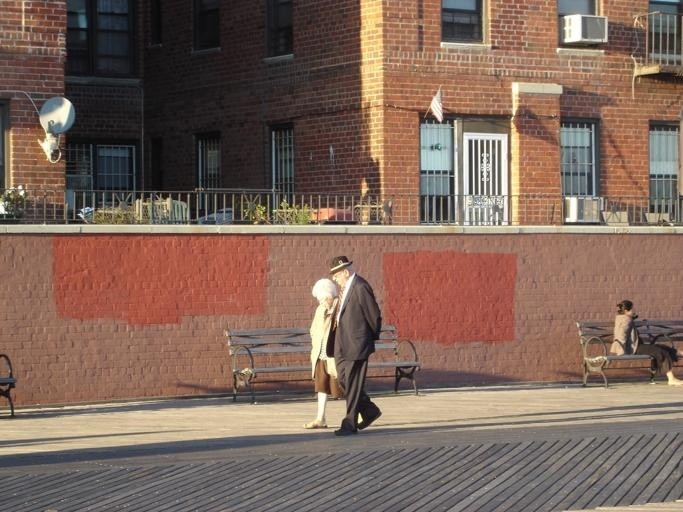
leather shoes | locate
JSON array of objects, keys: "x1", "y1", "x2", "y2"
[
  {"x1": 359, "y1": 410, "x2": 382, "y2": 430},
  {"x1": 335, "y1": 428, "x2": 358, "y2": 435}
]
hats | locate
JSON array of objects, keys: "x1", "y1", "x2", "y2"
[{"x1": 329, "y1": 256, "x2": 353, "y2": 274}]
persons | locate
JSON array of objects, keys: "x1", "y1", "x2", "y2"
[
  {"x1": 609, "y1": 298, "x2": 681, "y2": 389},
  {"x1": 322, "y1": 255, "x2": 381, "y2": 437},
  {"x1": 301, "y1": 279, "x2": 347, "y2": 431}
]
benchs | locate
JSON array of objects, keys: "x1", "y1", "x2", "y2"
[
  {"x1": 224, "y1": 324, "x2": 422, "y2": 405},
  {"x1": 576, "y1": 319, "x2": 683, "y2": 388},
  {"x1": 0, "y1": 354, "x2": 18, "y2": 417}
]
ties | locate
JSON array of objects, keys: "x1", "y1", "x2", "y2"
[{"x1": 331, "y1": 286, "x2": 345, "y2": 332}]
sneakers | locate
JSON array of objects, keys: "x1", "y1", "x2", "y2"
[
  {"x1": 669, "y1": 378, "x2": 682, "y2": 386},
  {"x1": 303, "y1": 420, "x2": 327, "y2": 428}
]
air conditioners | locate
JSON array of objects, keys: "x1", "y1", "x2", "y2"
[
  {"x1": 563, "y1": 195, "x2": 604, "y2": 224},
  {"x1": 560, "y1": 13, "x2": 609, "y2": 46}
]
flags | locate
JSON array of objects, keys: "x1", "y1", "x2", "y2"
[{"x1": 429, "y1": 90, "x2": 444, "y2": 122}]
